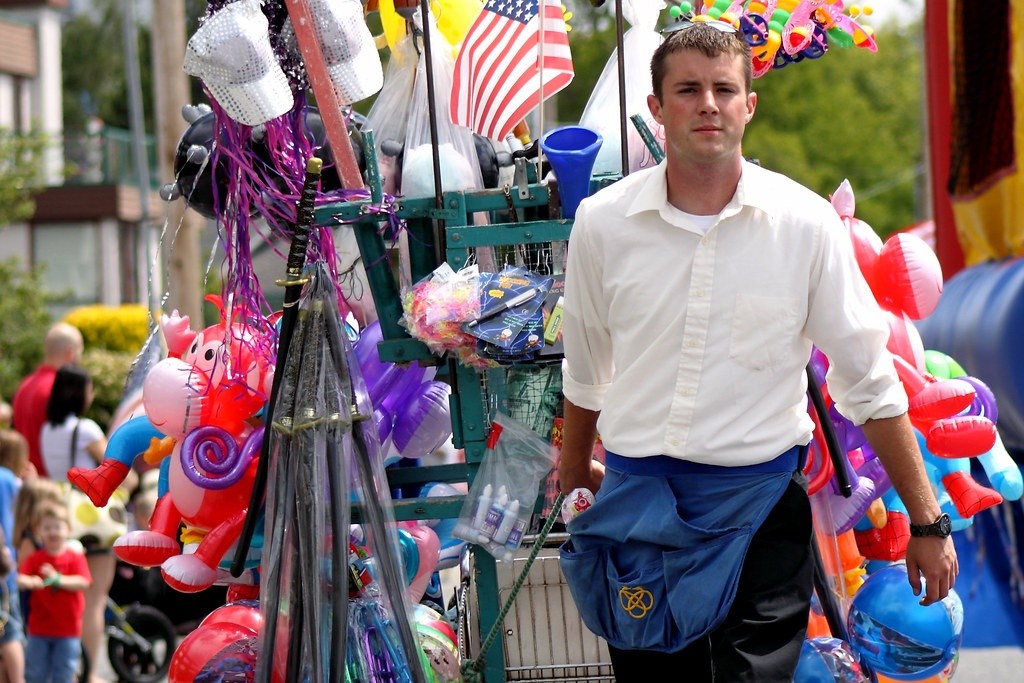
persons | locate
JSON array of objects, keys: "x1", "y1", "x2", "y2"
[
  {"x1": 556, "y1": 21, "x2": 959, "y2": 683},
  {"x1": 39, "y1": 362, "x2": 140, "y2": 683},
  {"x1": 12, "y1": 322, "x2": 84, "y2": 479},
  {"x1": 0, "y1": 397, "x2": 41, "y2": 683},
  {"x1": 15, "y1": 499, "x2": 93, "y2": 683}
]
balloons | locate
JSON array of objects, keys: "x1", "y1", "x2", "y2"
[
  {"x1": 157, "y1": 99, "x2": 362, "y2": 222},
  {"x1": 66, "y1": 295, "x2": 468, "y2": 683},
  {"x1": 800, "y1": 177, "x2": 1024, "y2": 683}
]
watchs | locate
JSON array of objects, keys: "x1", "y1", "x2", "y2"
[{"x1": 910, "y1": 513, "x2": 952, "y2": 539}]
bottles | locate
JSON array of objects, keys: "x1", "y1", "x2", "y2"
[{"x1": 453, "y1": 484, "x2": 528, "y2": 563}]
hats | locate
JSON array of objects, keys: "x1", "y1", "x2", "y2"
[
  {"x1": 282, "y1": 0, "x2": 383, "y2": 107},
  {"x1": 182, "y1": 0, "x2": 294, "y2": 126}
]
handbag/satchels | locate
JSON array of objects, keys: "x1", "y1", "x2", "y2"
[
  {"x1": 56, "y1": 423, "x2": 128, "y2": 557},
  {"x1": 558, "y1": 463, "x2": 796, "y2": 652}
]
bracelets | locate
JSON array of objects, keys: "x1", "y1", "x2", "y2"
[{"x1": 54, "y1": 572, "x2": 60, "y2": 588}]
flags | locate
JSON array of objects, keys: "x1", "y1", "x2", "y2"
[{"x1": 450, "y1": 0, "x2": 575, "y2": 143}]
